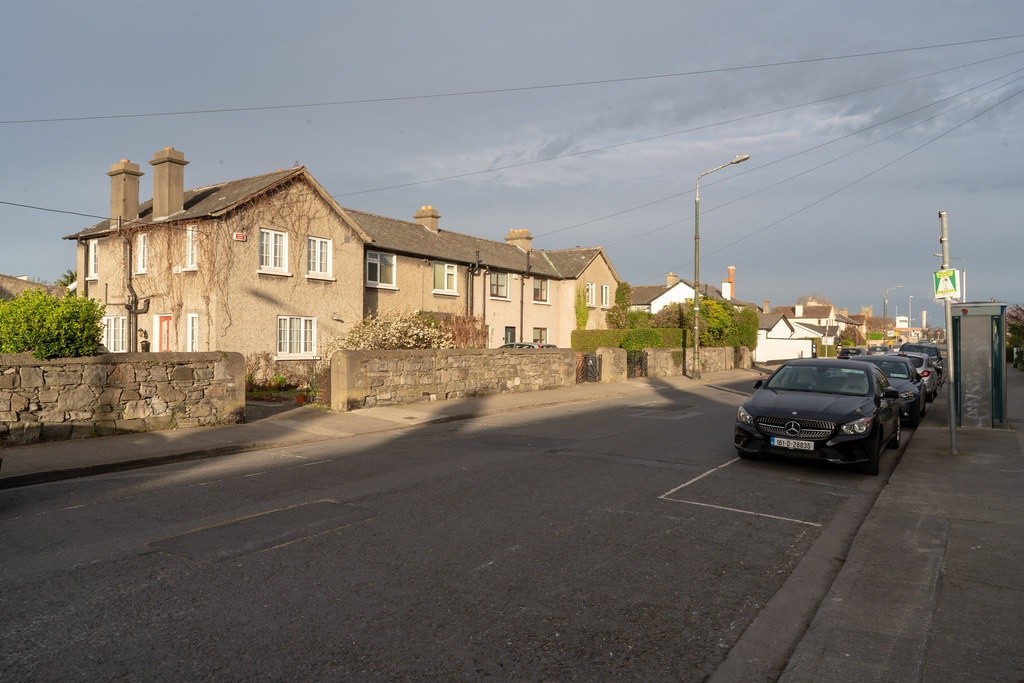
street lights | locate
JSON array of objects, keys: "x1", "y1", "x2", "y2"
[
  {"x1": 932, "y1": 251, "x2": 967, "y2": 302},
  {"x1": 693, "y1": 153, "x2": 751, "y2": 378},
  {"x1": 882, "y1": 285, "x2": 903, "y2": 340}
]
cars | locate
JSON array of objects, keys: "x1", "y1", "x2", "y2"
[
  {"x1": 733, "y1": 337, "x2": 944, "y2": 476},
  {"x1": 497, "y1": 342, "x2": 559, "y2": 351}
]
